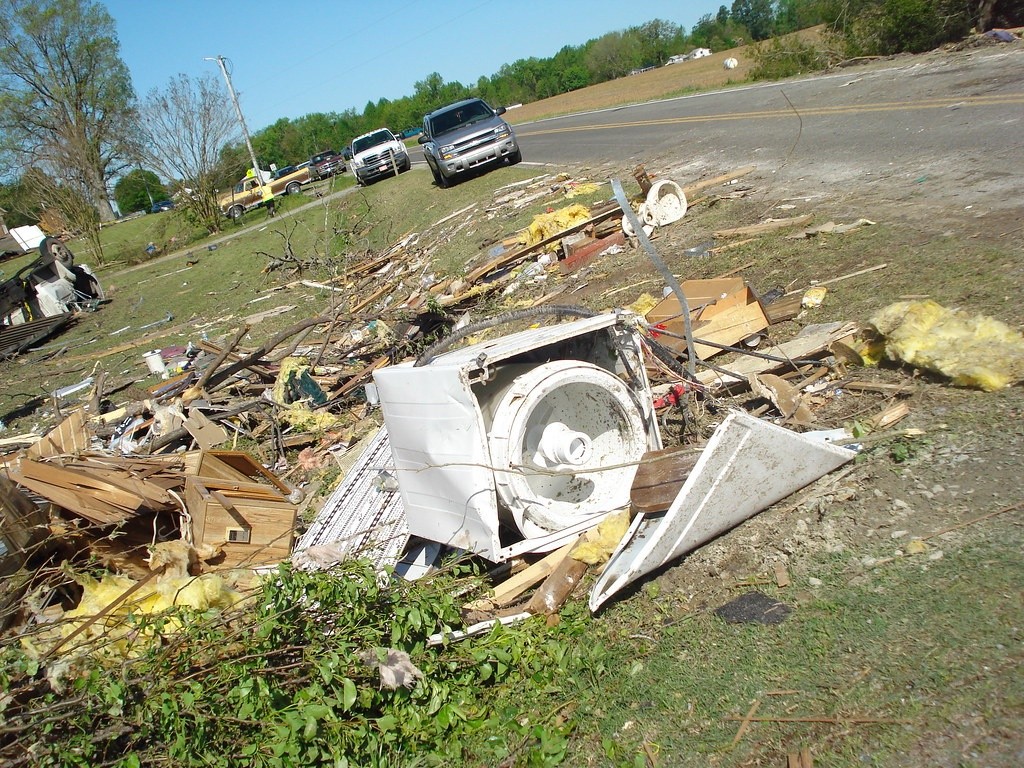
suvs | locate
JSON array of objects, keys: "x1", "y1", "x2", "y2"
[
  {"x1": 418, "y1": 98, "x2": 522, "y2": 187},
  {"x1": 399, "y1": 128, "x2": 423, "y2": 139}
]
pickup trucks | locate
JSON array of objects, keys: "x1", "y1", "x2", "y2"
[{"x1": 217, "y1": 167, "x2": 311, "y2": 219}]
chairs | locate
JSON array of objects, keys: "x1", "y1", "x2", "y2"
[
  {"x1": 356, "y1": 139, "x2": 372, "y2": 153},
  {"x1": 374, "y1": 133, "x2": 387, "y2": 144}
]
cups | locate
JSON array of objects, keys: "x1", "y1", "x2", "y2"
[{"x1": 142, "y1": 349, "x2": 165, "y2": 374}]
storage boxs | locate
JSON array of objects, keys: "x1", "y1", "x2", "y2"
[{"x1": 373, "y1": 314, "x2": 664, "y2": 565}]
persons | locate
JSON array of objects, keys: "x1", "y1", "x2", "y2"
[{"x1": 261, "y1": 182, "x2": 275, "y2": 218}]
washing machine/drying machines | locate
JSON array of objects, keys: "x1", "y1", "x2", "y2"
[{"x1": 371, "y1": 312, "x2": 664, "y2": 563}]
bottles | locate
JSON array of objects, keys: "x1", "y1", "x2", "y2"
[{"x1": 202, "y1": 331, "x2": 208, "y2": 341}]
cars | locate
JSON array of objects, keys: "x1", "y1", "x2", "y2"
[
  {"x1": 344, "y1": 128, "x2": 411, "y2": 185},
  {"x1": 184, "y1": 189, "x2": 220, "y2": 203},
  {"x1": 151, "y1": 201, "x2": 176, "y2": 213},
  {"x1": 274, "y1": 166, "x2": 298, "y2": 180},
  {"x1": 295, "y1": 161, "x2": 310, "y2": 171},
  {"x1": 307, "y1": 150, "x2": 347, "y2": 181},
  {"x1": 0, "y1": 236, "x2": 105, "y2": 325}
]
